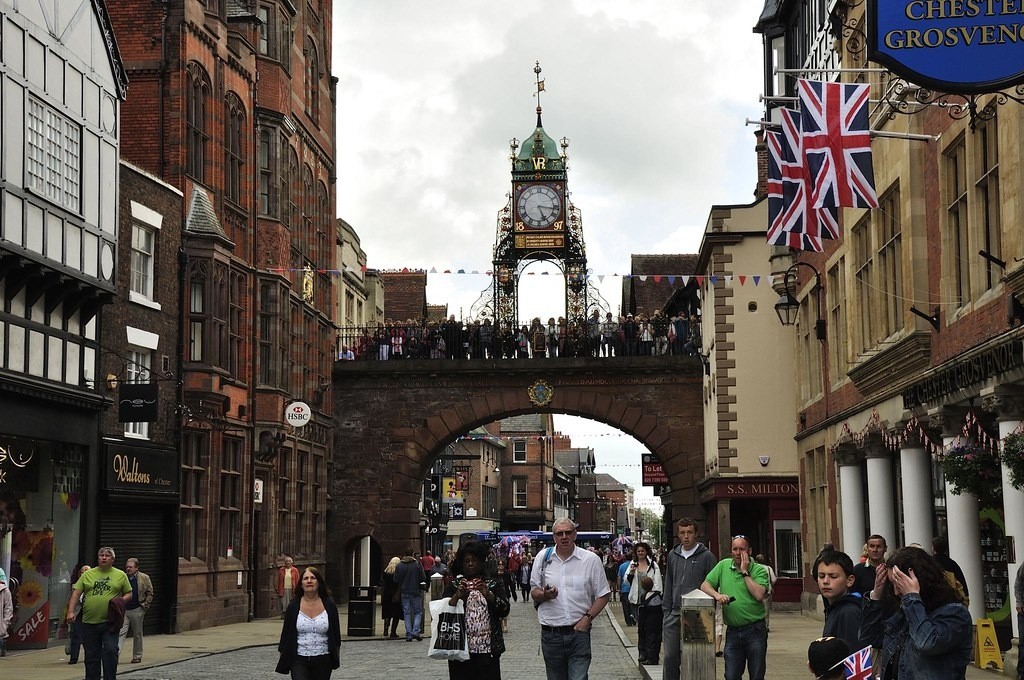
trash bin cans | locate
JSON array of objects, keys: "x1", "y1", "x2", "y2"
[{"x1": 347, "y1": 586, "x2": 379, "y2": 637}]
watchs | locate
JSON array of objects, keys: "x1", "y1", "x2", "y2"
[
  {"x1": 586, "y1": 612, "x2": 594, "y2": 620},
  {"x1": 742, "y1": 571, "x2": 750, "y2": 578}
]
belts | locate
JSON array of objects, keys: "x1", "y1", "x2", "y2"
[{"x1": 541, "y1": 624, "x2": 574, "y2": 633}]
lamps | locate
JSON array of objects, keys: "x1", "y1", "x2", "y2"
[{"x1": 775, "y1": 262, "x2": 826, "y2": 341}]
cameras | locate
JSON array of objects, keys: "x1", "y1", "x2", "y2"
[{"x1": 466, "y1": 581, "x2": 477, "y2": 589}]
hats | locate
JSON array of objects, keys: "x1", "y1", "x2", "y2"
[{"x1": 435, "y1": 557, "x2": 441, "y2": 561}]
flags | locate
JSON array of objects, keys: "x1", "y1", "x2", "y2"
[
  {"x1": 766, "y1": 130, "x2": 824, "y2": 253},
  {"x1": 780, "y1": 107, "x2": 840, "y2": 241},
  {"x1": 798, "y1": 78, "x2": 879, "y2": 208}
]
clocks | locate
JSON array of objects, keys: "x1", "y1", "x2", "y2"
[{"x1": 518, "y1": 185, "x2": 561, "y2": 226}]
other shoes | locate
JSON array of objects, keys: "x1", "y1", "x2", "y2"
[
  {"x1": 716, "y1": 651, "x2": 722, "y2": 656},
  {"x1": 415, "y1": 637, "x2": 423, "y2": 641},
  {"x1": 390, "y1": 630, "x2": 400, "y2": 637},
  {"x1": 626, "y1": 614, "x2": 636, "y2": 627},
  {"x1": 131, "y1": 658, "x2": 141, "y2": 663},
  {"x1": 765, "y1": 624, "x2": 769, "y2": 632},
  {"x1": 608, "y1": 597, "x2": 612, "y2": 602},
  {"x1": 406, "y1": 638, "x2": 412, "y2": 642},
  {"x1": 501, "y1": 617, "x2": 509, "y2": 633},
  {"x1": 384, "y1": 630, "x2": 388, "y2": 636},
  {"x1": 68, "y1": 659, "x2": 77, "y2": 664},
  {"x1": 638, "y1": 654, "x2": 646, "y2": 662},
  {"x1": 613, "y1": 595, "x2": 617, "y2": 602},
  {"x1": 281, "y1": 612, "x2": 286, "y2": 619}
]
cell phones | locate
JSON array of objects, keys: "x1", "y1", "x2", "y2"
[{"x1": 727, "y1": 596, "x2": 736, "y2": 603}]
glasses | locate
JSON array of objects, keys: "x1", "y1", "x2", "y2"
[
  {"x1": 554, "y1": 529, "x2": 575, "y2": 536},
  {"x1": 734, "y1": 535, "x2": 746, "y2": 539}
]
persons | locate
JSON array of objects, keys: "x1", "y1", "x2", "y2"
[
  {"x1": 527, "y1": 553, "x2": 535, "y2": 571},
  {"x1": 275, "y1": 557, "x2": 300, "y2": 619},
  {"x1": 67, "y1": 560, "x2": 91, "y2": 664},
  {"x1": 492, "y1": 559, "x2": 517, "y2": 632},
  {"x1": 339, "y1": 309, "x2": 703, "y2": 361},
  {"x1": 0, "y1": 568, "x2": 13, "y2": 640},
  {"x1": 414, "y1": 549, "x2": 448, "y2": 592},
  {"x1": 530, "y1": 517, "x2": 611, "y2": 680},
  {"x1": 661, "y1": 517, "x2": 1024, "y2": 680},
  {"x1": 381, "y1": 547, "x2": 427, "y2": 641},
  {"x1": 118, "y1": 558, "x2": 154, "y2": 663},
  {"x1": 517, "y1": 557, "x2": 532, "y2": 602},
  {"x1": 275, "y1": 566, "x2": 341, "y2": 680},
  {"x1": 442, "y1": 541, "x2": 510, "y2": 680},
  {"x1": 67, "y1": 547, "x2": 133, "y2": 680},
  {"x1": 491, "y1": 543, "x2": 526, "y2": 589},
  {"x1": 587, "y1": 543, "x2": 668, "y2": 626}
]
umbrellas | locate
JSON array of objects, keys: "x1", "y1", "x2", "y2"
[
  {"x1": 611, "y1": 536, "x2": 634, "y2": 544},
  {"x1": 500, "y1": 536, "x2": 521, "y2": 543}
]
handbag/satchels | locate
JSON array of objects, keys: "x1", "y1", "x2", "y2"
[
  {"x1": 428, "y1": 597, "x2": 470, "y2": 663},
  {"x1": 65, "y1": 622, "x2": 72, "y2": 654},
  {"x1": 418, "y1": 561, "x2": 431, "y2": 590},
  {"x1": 392, "y1": 591, "x2": 402, "y2": 608}
]
